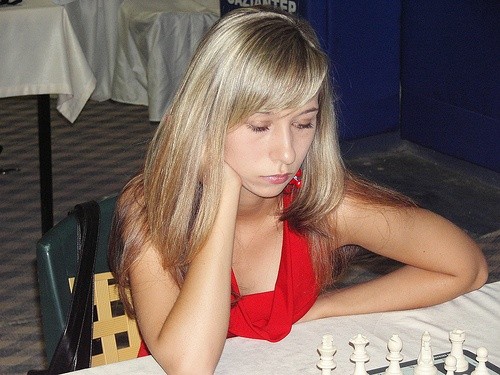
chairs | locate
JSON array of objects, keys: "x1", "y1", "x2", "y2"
[{"x1": 36, "y1": 191, "x2": 148, "y2": 373}]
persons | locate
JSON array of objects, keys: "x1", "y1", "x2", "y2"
[{"x1": 112, "y1": 3, "x2": 489, "y2": 375}]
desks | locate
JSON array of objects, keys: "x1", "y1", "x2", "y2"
[
  {"x1": 61, "y1": 275, "x2": 500, "y2": 375},
  {"x1": 1, "y1": 0, "x2": 222, "y2": 240}
]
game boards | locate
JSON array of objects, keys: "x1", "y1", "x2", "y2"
[{"x1": 369, "y1": 348, "x2": 500, "y2": 374}]
